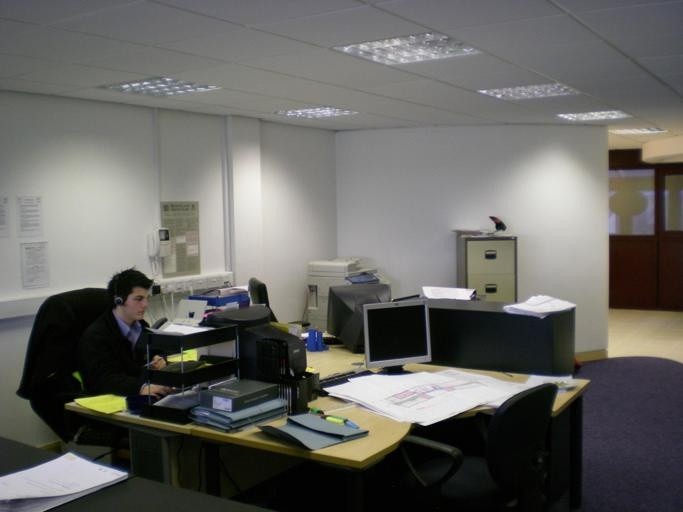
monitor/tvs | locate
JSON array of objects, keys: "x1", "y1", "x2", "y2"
[
  {"x1": 206, "y1": 305, "x2": 307, "y2": 386},
  {"x1": 363, "y1": 299, "x2": 432, "y2": 375}
]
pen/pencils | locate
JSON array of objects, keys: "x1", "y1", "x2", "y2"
[{"x1": 321, "y1": 414, "x2": 358, "y2": 430}]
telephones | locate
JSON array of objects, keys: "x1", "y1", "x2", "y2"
[{"x1": 147, "y1": 228, "x2": 172, "y2": 258}]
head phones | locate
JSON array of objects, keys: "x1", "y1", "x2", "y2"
[{"x1": 113, "y1": 276, "x2": 124, "y2": 305}]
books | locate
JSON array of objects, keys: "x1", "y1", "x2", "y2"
[
  {"x1": 526, "y1": 374, "x2": 578, "y2": 391},
  {"x1": 0, "y1": 451, "x2": 128, "y2": 512},
  {"x1": 503, "y1": 295, "x2": 577, "y2": 319},
  {"x1": 188, "y1": 395, "x2": 288, "y2": 435}
]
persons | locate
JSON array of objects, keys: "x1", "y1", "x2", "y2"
[{"x1": 71, "y1": 263, "x2": 172, "y2": 482}]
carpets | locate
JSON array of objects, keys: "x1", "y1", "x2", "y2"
[{"x1": 576, "y1": 356, "x2": 683, "y2": 512}]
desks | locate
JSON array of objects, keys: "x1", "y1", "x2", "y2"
[{"x1": 0, "y1": 299, "x2": 591, "y2": 512}]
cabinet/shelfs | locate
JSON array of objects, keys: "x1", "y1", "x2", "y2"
[{"x1": 455, "y1": 236, "x2": 518, "y2": 302}]
